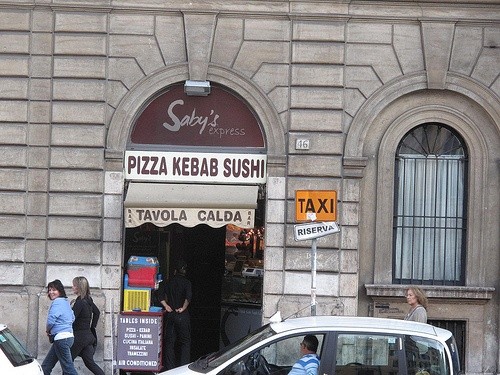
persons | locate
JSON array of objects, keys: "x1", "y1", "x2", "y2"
[
  {"x1": 288, "y1": 335, "x2": 320, "y2": 375},
  {"x1": 156, "y1": 265, "x2": 192, "y2": 370},
  {"x1": 63, "y1": 277, "x2": 105, "y2": 375},
  {"x1": 404, "y1": 287, "x2": 430, "y2": 370},
  {"x1": 41, "y1": 280, "x2": 78, "y2": 375}
]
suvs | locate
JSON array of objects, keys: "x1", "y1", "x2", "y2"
[{"x1": 242, "y1": 268, "x2": 264, "y2": 278}]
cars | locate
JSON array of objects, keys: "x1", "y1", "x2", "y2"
[
  {"x1": 153, "y1": 316, "x2": 461, "y2": 375},
  {"x1": 0, "y1": 323, "x2": 43, "y2": 374}
]
